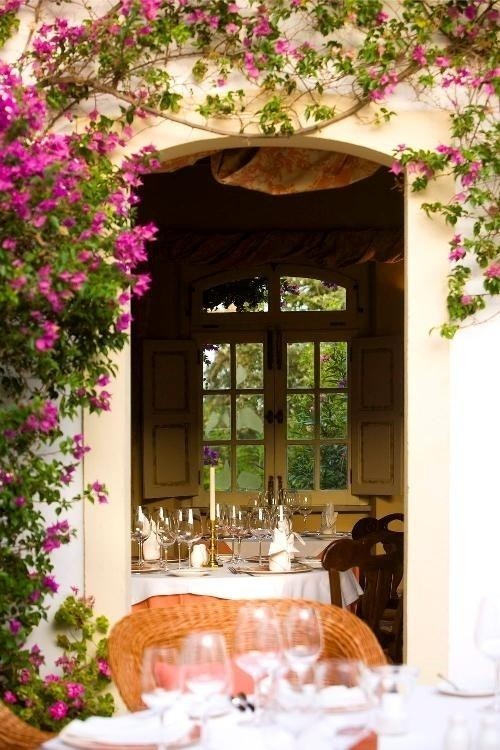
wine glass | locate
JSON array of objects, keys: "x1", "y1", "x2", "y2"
[
  {"x1": 232, "y1": 603, "x2": 282, "y2": 697},
  {"x1": 282, "y1": 605, "x2": 325, "y2": 691},
  {"x1": 140, "y1": 645, "x2": 182, "y2": 750},
  {"x1": 131, "y1": 489, "x2": 313, "y2": 573}
]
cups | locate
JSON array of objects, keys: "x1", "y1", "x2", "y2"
[{"x1": 184, "y1": 630, "x2": 230, "y2": 703}]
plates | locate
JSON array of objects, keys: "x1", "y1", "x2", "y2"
[
  {"x1": 303, "y1": 528, "x2": 349, "y2": 542},
  {"x1": 169, "y1": 567, "x2": 211, "y2": 577}
]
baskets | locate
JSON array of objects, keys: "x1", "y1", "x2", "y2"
[{"x1": 0, "y1": 693, "x2": 64, "y2": 743}]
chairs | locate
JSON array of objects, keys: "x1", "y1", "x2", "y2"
[
  {"x1": 321, "y1": 513, "x2": 404, "y2": 663},
  {"x1": 107, "y1": 598, "x2": 390, "y2": 714}
]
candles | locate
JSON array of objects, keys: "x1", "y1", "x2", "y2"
[{"x1": 209, "y1": 464, "x2": 216, "y2": 522}]
